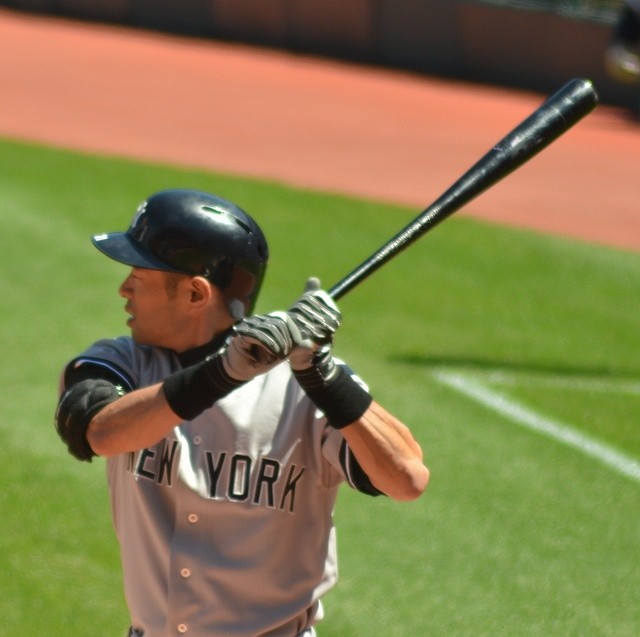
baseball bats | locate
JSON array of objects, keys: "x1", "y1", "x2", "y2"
[{"x1": 330, "y1": 79, "x2": 599, "y2": 304}]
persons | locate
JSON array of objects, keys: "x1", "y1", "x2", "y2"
[{"x1": 52, "y1": 185, "x2": 431, "y2": 633}]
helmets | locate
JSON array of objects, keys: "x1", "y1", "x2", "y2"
[{"x1": 91, "y1": 189, "x2": 269, "y2": 316}]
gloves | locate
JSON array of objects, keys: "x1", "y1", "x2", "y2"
[
  {"x1": 163, "y1": 311, "x2": 303, "y2": 420},
  {"x1": 286, "y1": 276, "x2": 373, "y2": 428}
]
